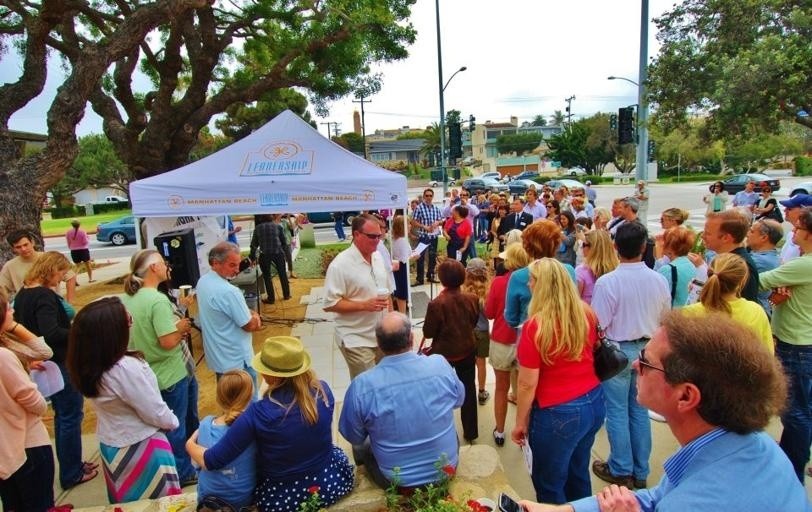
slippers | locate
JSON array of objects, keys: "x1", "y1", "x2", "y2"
[
  {"x1": 507, "y1": 391, "x2": 518, "y2": 406},
  {"x1": 75, "y1": 279, "x2": 97, "y2": 286}
]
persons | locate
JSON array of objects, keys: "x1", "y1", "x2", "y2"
[{"x1": 0, "y1": 172, "x2": 812, "y2": 512}]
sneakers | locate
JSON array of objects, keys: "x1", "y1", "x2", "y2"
[
  {"x1": 338, "y1": 234, "x2": 347, "y2": 242},
  {"x1": 179, "y1": 470, "x2": 199, "y2": 487},
  {"x1": 593, "y1": 459, "x2": 634, "y2": 492},
  {"x1": 477, "y1": 389, "x2": 490, "y2": 405},
  {"x1": 411, "y1": 280, "x2": 424, "y2": 287},
  {"x1": 426, "y1": 278, "x2": 440, "y2": 283},
  {"x1": 261, "y1": 274, "x2": 297, "y2": 304},
  {"x1": 493, "y1": 428, "x2": 505, "y2": 447},
  {"x1": 633, "y1": 476, "x2": 647, "y2": 490}
]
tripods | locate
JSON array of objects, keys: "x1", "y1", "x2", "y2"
[
  {"x1": 255, "y1": 259, "x2": 289, "y2": 325},
  {"x1": 180, "y1": 290, "x2": 205, "y2": 369}
]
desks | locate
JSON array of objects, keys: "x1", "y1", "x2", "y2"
[{"x1": 229, "y1": 264, "x2": 266, "y2": 295}]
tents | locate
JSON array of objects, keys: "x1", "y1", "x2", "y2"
[{"x1": 130, "y1": 108, "x2": 412, "y2": 319}]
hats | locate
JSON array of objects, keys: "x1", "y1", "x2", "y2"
[
  {"x1": 778, "y1": 193, "x2": 812, "y2": 208},
  {"x1": 250, "y1": 335, "x2": 312, "y2": 378}
]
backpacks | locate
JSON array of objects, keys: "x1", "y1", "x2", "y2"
[{"x1": 766, "y1": 199, "x2": 784, "y2": 224}]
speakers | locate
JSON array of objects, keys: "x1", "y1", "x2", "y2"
[{"x1": 153, "y1": 228, "x2": 199, "y2": 290}]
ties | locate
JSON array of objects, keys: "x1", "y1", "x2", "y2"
[{"x1": 515, "y1": 213, "x2": 520, "y2": 229}]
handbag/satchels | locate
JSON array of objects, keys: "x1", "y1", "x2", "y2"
[{"x1": 592, "y1": 337, "x2": 628, "y2": 382}]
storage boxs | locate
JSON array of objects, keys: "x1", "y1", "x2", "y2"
[{"x1": 242, "y1": 288, "x2": 261, "y2": 312}]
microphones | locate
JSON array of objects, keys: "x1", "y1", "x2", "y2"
[{"x1": 250, "y1": 245, "x2": 265, "y2": 256}]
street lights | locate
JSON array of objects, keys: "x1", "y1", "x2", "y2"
[
  {"x1": 439, "y1": 66, "x2": 468, "y2": 198},
  {"x1": 605, "y1": 75, "x2": 648, "y2": 190}
]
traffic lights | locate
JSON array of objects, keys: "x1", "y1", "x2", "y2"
[
  {"x1": 610, "y1": 114, "x2": 617, "y2": 129},
  {"x1": 648, "y1": 139, "x2": 655, "y2": 156},
  {"x1": 469, "y1": 114, "x2": 476, "y2": 133}
]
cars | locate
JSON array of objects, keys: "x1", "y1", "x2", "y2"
[
  {"x1": 542, "y1": 178, "x2": 597, "y2": 203},
  {"x1": 103, "y1": 196, "x2": 129, "y2": 205},
  {"x1": 96, "y1": 213, "x2": 137, "y2": 248},
  {"x1": 460, "y1": 167, "x2": 587, "y2": 195},
  {"x1": 305, "y1": 211, "x2": 360, "y2": 227},
  {"x1": 705, "y1": 171, "x2": 782, "y2": 195},
  {"x1": 787, "y1": 180, "x2": 812, "y2": 198},
  {"x1": 429, "y1": 176, "x2": 457, "y2": 187}
]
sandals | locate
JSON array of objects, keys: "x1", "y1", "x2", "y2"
[
  {"x1": 82, "y1": 460, "x2": 100, "y2": 471},
  {"x1": 72, "y1": 468, "x2": 100, "y2": 487}
]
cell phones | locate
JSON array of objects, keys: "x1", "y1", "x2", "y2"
[{"x1": 498, "y1": 493, "x2": 527, "y2": 512}]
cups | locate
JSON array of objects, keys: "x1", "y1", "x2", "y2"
[
  {"x1": 392, "y1": 259, "x2": 400, "y2": 270},
  {"x1": 180, "y1": 285, "x2": 192, "y2": 298},
  {"x1": 474, "y1": 496, "x2": 497, "y2": 512},
  {"x1": 376, "y1": 288, "x2": 389, "y2": 308}
]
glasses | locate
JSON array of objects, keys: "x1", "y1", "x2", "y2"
[
  {"x1": 546, "y1": 206, "x2": 554, "y2": 209},
  {"x1": 359, "y1": 231, "x2": 383, "y2": 239},
  {"x1": 582, "y1": 242, "x2": 592, "y2": 249},
  {"x1": 639, "y1": 347, "x2": 666, "y2": 377}
]
